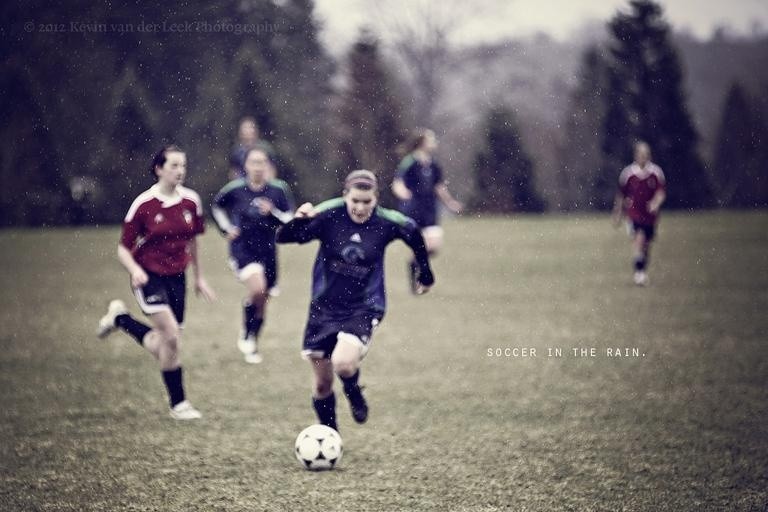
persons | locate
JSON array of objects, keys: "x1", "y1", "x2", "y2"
[
  {"x1": 389, "y1": 125, "x2": 464, "y2": 296},
  {"x1": 93, "y1": 142, "x2": 219, "y2": 423},
  {"x1": 274, "y1": 168, "x2": 434, "y2": 430},
  {"x1": 225, "y1": 113, "x2": 289, "y2": 182},
  {"x1": 208, "y1": 146, "x2": 298, "y2": 366},
  {"x1": 608, "y1": 137, "x2": 669, "y2": 289}
]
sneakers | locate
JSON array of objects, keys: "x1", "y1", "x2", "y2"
[
  {"x1": 94, "y1": 299, "x2": 129, "y2": 341},
  {"x1": 633, "y1": 271, "x2": 648, "y2": 286},
  {"x1": 342, "y1": 384, "x2": 369, "y2": 424},
  {"x1": 167, "y1": 399, "x2": 203, "y2": 423},
  {"x1": 237, "y1": 330, "x2": 264, "y2": 364}
]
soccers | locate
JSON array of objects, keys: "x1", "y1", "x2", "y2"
[{"x1": 296, "y1": 422, "x2": 343, "y2": 470}]
